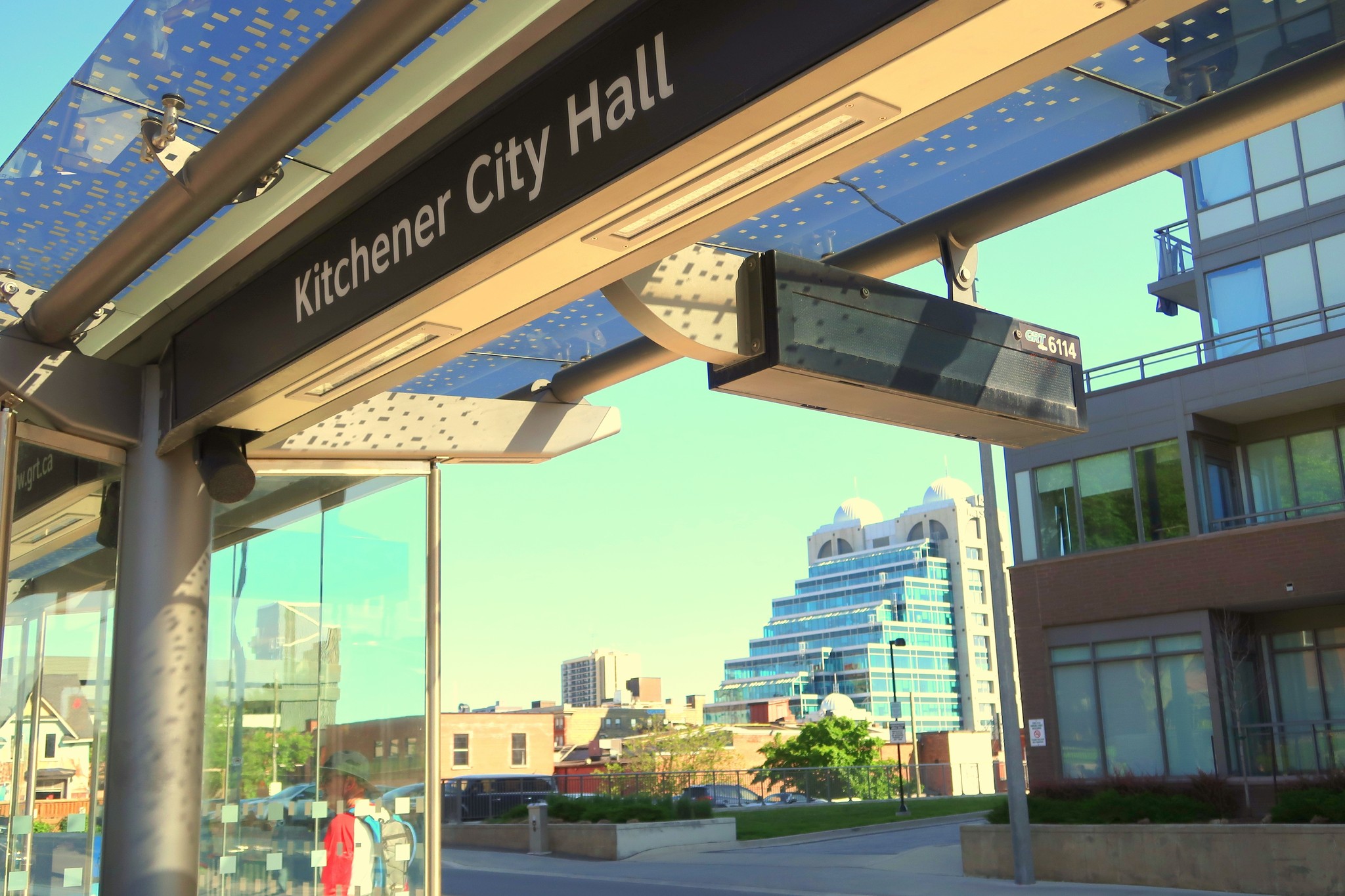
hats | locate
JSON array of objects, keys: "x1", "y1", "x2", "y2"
[{"x1": 320, "y1": 749, "x2": 383, "y2": 798}]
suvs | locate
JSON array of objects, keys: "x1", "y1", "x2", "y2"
[{"x1": 669, "y1": 783, "x2": 763, "y2": 807}]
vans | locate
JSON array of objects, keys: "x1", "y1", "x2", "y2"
[{"x1": 444, "y1": 774, "x2": 559, "y2": 822}]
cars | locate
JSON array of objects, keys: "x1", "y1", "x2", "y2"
[
  {"x1": 744, "y1": 792, "x2": 815, "y2": 806},
  {"x1": 202, "y1": 782, "x2": 446, "y2": 827}
]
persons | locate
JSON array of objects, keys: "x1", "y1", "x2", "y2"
[{"x1": 314, "y1": 750, "x2": 409, "y2": 896}]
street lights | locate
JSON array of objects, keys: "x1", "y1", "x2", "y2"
[{"x1": 888, "y1": 638, "x2": 908, "y2": 812}]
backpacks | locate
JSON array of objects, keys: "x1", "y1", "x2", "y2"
[{"x1": 345, "y1": 804, "x2": 418, "y2": 896}]
